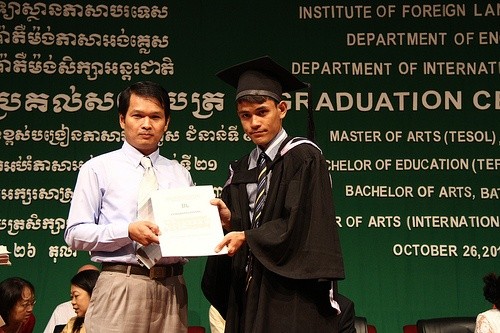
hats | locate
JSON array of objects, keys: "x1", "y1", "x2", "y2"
[{"x1": 216, "y1": 56, "x2": 317, "y2": 143}]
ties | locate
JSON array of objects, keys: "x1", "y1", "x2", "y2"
[{"x1": 245, "y1": 152, "x2": 268, "y2": 291}]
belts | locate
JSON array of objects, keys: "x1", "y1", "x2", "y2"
[{"x1": 102, "y1": 262, "x2": 183, "y2": 279}]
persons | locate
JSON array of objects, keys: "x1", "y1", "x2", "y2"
[
  {"x1": 42, "y1": 265, "x2": 101, "y2": 333},
  {"x1": 209, "y1": 294, "x2": 356, "y2": 333},
  {"x1": 474, "y1": 273, "x2": 500, "y2": 333},
  {"x1": 64, "y1": 81, "x2": 196, "y2": 333},
  {"x1": 0, "y1": 277, "x2": 36, "y2": 333},
  {"x1": 201, "y1": 56, "x2": 345, "y2": 333}
]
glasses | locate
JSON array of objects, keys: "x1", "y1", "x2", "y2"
[{"x1": 15, "y1": 298, "x2": 36, "y2": 308}]
